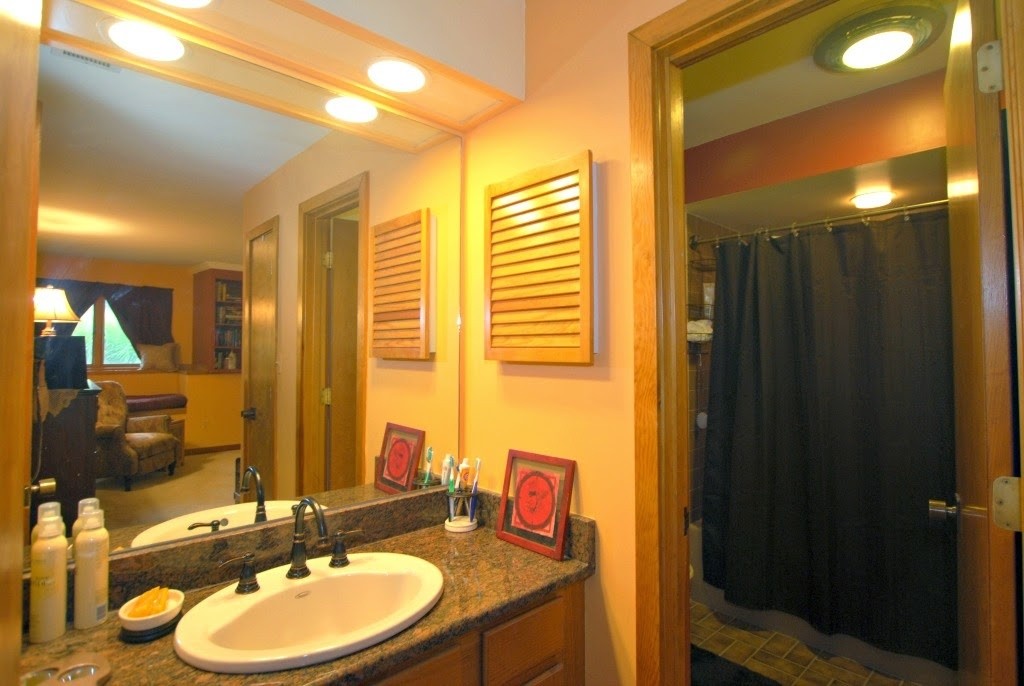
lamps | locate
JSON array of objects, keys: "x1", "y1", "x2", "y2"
[{"x1": 32, "y1": 283, "x2": 81, "y2": 337}]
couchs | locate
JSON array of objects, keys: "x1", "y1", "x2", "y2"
[{"x1": 89, "y1": 381, "x2": 180, "y2": 492}]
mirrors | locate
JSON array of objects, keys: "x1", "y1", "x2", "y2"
[{"x1": 23, "y1": 2, "x2": 467, "y2": 573}]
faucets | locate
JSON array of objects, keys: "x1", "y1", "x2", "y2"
[
  {"x1": 240, "y1": 466, "x2": 268, "y2": 524},
  {"x1": 286, "y1": 496, "x2": 329, "y2": 579}
]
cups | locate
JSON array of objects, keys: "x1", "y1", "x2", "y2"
[{"x1": 445, "y1": 487, "x2": 479, "y2": 532}]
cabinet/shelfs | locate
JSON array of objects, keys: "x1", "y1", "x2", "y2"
[
  {"x1": 189, "y1": 269, "x2": 242, "y2": 375},
  {"x1": 685, "y1": 258, "x2": 720, "y2": 343},
  {"x1": 38, "y1": 378, "x2": 105, "y2": 538},
  {"x1": 361, "y1": 586, "x2": 588, "y2": 686}
]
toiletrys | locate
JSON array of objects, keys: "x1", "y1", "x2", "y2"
[
  {"x1": 453, "y1": 457, "x2": 471, "y2": 522},
  {"x1": 31, "y1": 497, "x2": 100, "y2": 569},
  {"x1": 441, "y1": 454, "x2": 453, "y2": 486},
  {"x1": 28, "y1": 509, "x2": 110, "y2": 645}
]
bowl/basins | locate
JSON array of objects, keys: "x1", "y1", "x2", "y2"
[{"x1": 120, "y1": 586, "x2": 186, "y2": 631}]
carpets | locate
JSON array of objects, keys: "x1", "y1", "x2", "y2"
[{"x1": 691, "y1": 640, "x2": 782, "y2": 686}]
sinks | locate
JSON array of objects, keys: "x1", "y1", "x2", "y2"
[
  {"x1": 129, "y1": 499, "x2": 330, "y2": 548},
  {"x1": 172, "y1": 551, "x2": 445, "y2": 675}
]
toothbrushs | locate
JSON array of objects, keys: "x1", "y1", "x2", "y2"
[
  {"x1": 447, "y1": 455, "x2": 481, "y2": 523},
  {"x1": 420, "y1": 446, "x2": 434, "y2": 488}
]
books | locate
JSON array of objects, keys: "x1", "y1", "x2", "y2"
[{"x1": 209, "y1": 275, "x2": 243, "y2": 373}]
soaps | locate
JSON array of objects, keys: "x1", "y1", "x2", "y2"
[{"x1": 127, "y1": 586, "x2": 169, "y2": 619}]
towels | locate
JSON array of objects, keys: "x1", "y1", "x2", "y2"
[{"x1": 687, "y1": 319, "x2": 715, "y2": 344}]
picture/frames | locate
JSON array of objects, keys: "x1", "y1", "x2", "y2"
[
  {"x1": 496, "y1": 447, "x2": 575, "y2": 561},
  {"x1": 374, "y1": 422, "x2": 425, "y2": 496}
]
